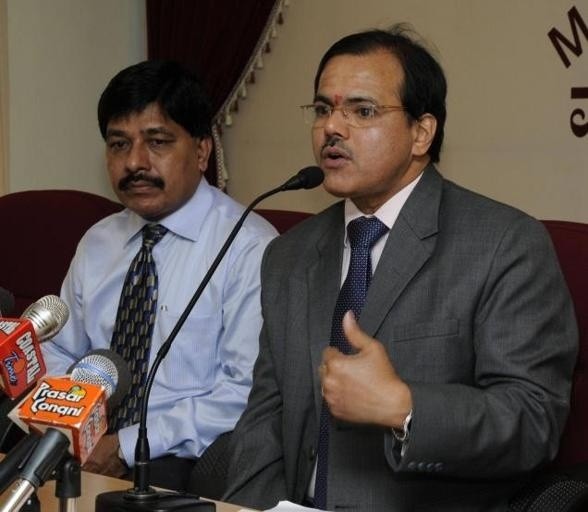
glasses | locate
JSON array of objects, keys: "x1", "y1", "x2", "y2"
[{"x1": 295, "y1": 103, "x2": 407, "y2": 126}]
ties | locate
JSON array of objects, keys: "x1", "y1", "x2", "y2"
[
  {"x1": 313, "y1": 216, "x2": 388, "y2": 511},
  {"x1": 103, "y1": 222, "x2": 169, "y2": 435}
]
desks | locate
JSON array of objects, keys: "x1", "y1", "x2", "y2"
[{"x1": 1, "y1": 453, "x2": 253, "y2": 510}]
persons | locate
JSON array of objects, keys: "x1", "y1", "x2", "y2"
[
  {"x1": 37, "y1": 58, "x2": 280, "y2": 485},
  {"x1": 219, "y1": 30, "x2": 580, "y2": 512}
]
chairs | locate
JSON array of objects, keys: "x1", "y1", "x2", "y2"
[
  {"x1": 539, "y1": 219, "x2": 588, "y2": 466},
  {"x1": 2, "y1": 190, "x2": 126, "y2": 318},
  {"x1": 252, "y1": 209, "x2": 315, "y2": 234}
]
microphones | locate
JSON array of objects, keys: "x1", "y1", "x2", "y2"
[
  {"x1": 0, "y1": 294, "x2": 70, "y2": 400},
  {"x1": 0, "y1": 286, "x2": 18, "y2": 318},
  {"x1": 0, "y1": 348, "x2": 131, "y2": 496},
  {"x1": 94, "y1": 166, "x2": 325, "y2": 512},
  {"x1": 0, "y1": 354, "x2": 119, "y2": 512}
]
